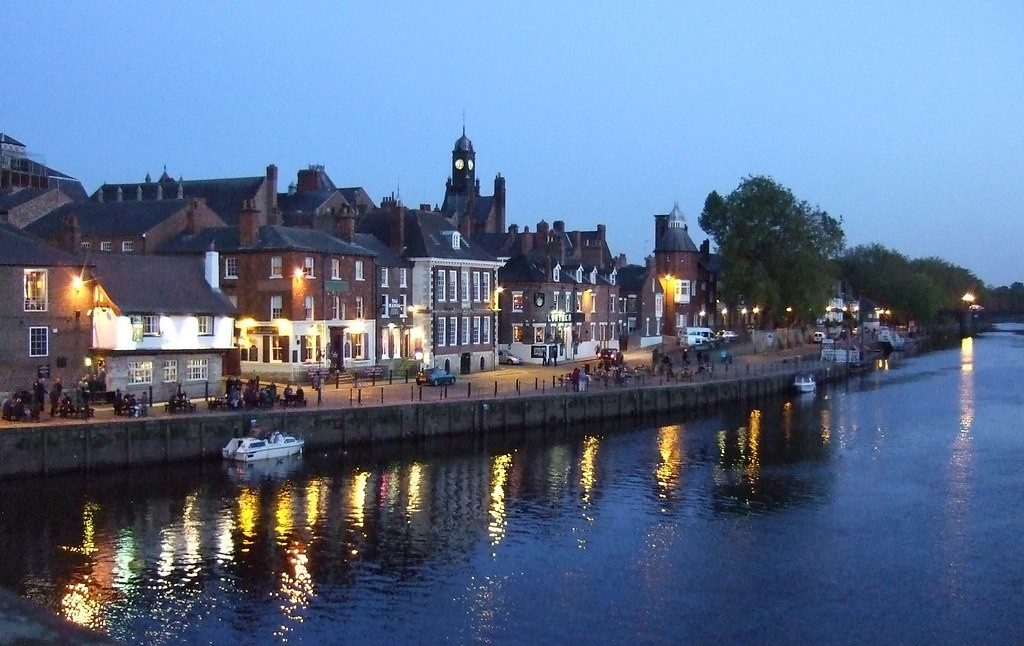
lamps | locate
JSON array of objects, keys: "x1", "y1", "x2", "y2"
[
  {"x1": 494, "y1": 286, "x2": 504, "y2": 294},
  {"x1": 85, "y1": 357, "x2": 92, "y2": 367},
  {"x1": 290, "y1": 266, "x2": 303, "y2": 284},
  {"x1": 664, "y1": 275, "x2": 671, "y2": 281},
  {"x1": 584, "y1": 288, "x2": 593, "y2": 294},
  {"x1": 72, "y1": 276, "x2": 83, "y2": 293}
]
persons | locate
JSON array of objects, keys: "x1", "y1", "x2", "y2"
[
  {"x1": 579, "y1": 368, "x2": 586, "y2": 392},
  {"x1": 552, "y1": 348, "x2": 557, "y2": 366},
  {"x1": 542, "y1": 349, "x2": 546, "y2": 366},
  {"x1": 597, "y1": 360, "x2": 604, "y2": 368},
  {"x1": 696, "y1": 348, "x2": 704, "y2": 369},
  {"x1": 682, "y1": 348, "x2": 688, "y2": 366},
  {"x1": 605, "y1": 361, "x2": 611, "y2": 371},
  {"x1": 571, "y1": 367, "x2": 579, "y2": 392},
  {"x1": 651, "y1": 347, "x2": 658, "y2": 376},
  {"x1": 703, "y1": 349, "x2": 710, "y2": 370},
  {"x1": 3, "y1": 361, "x2": 304, "y2": 422}
]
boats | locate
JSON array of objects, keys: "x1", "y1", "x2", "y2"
[
  {"x1": 792, "y1": 374, "x2": 819, "y2": 393},
  {"x1": 220, "y1": 427, "x2": 306, "y2": 461}
]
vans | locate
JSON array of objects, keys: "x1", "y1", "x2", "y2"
[
  {"x1": 675, "y1": 327, "x2": 721, "y2": 347},
  {"x1": 812, "y1": 330, "x2": 826, "y2": 344}
]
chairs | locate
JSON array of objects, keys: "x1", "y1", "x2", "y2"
[{"x1": 2, "y1": 393, "x2": 308, "y2": 423}]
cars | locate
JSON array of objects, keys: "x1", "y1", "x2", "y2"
[
  {"x1": 417, "y1": 368, "x2": 456, "y2": 387},
  {"x1": 718, "y1": 330, "x2": 739, "y2": 343},
  {"x1": 498, "y1": 347, "x2": 523, "y2": 366},
  {"x1": 679, "y1": 335, "x2": 711, "y2": 350},
  {"x1": 596, "y1": 347, "x2": 623, "y2": 364}
]
